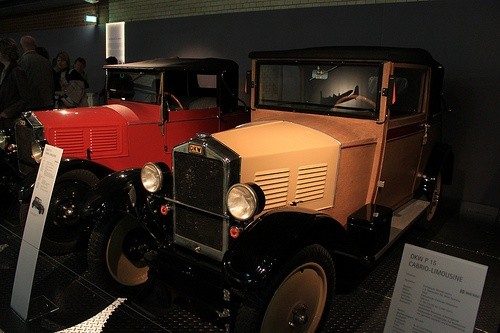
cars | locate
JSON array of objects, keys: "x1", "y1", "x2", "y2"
[
  {"x1": 86, "y1": 43, "x2": 448, "y2": 333},
  {"x1": 31, "y1": 196, "x2": 45, "y2": 214},
  {"x1": 0, "y1": 55, "x2": 251, "y2": 250}
]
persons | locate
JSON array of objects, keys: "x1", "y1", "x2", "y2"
[
  {"x1": 0, "y1": 35, "x2": 90, "y2": 127},
  {"x1": 99, "y1": 57, "x2": 134, "y2": 101}
]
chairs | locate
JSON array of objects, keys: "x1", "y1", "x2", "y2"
[{"x1": 365, "y1": 75, "x2": 408, "y2": 112}]
